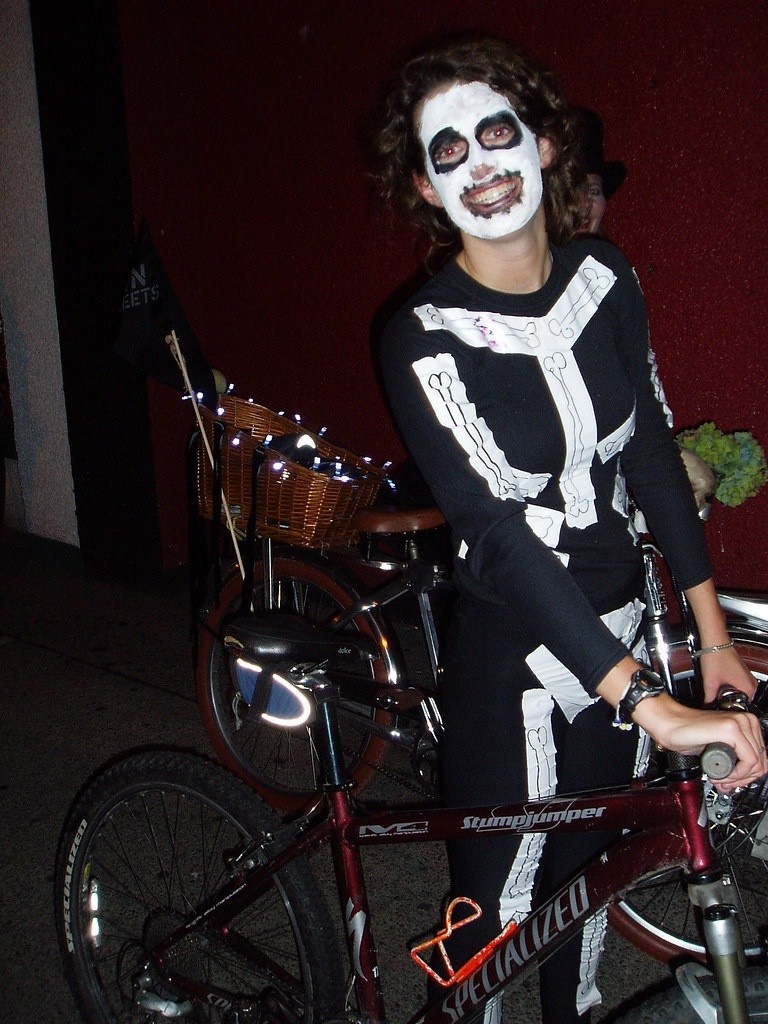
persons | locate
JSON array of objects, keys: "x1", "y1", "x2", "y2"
[{"x1": 363, "y1": 35, "x2": 768, "y2": 1024}]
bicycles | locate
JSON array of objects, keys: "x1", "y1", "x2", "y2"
[{"x1": 57, "y1": 391, "x2": 768, "y2": 1024}]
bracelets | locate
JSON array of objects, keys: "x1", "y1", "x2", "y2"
[{"x1": 695, "y1": 641, "x2": 736, "y2": 655}]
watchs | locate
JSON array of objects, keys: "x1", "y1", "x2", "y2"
[{"x1": 619, "y1": 667, "x2": 665, "y2": 725}]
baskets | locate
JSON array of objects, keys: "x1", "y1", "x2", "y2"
[{"x1": 193, "y1": 394, "x2": 386, "y2": 550}]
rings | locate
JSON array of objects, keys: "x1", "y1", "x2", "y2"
[{"x1": 761, "y1": 746, "x2": 764, "y2": 750}]
